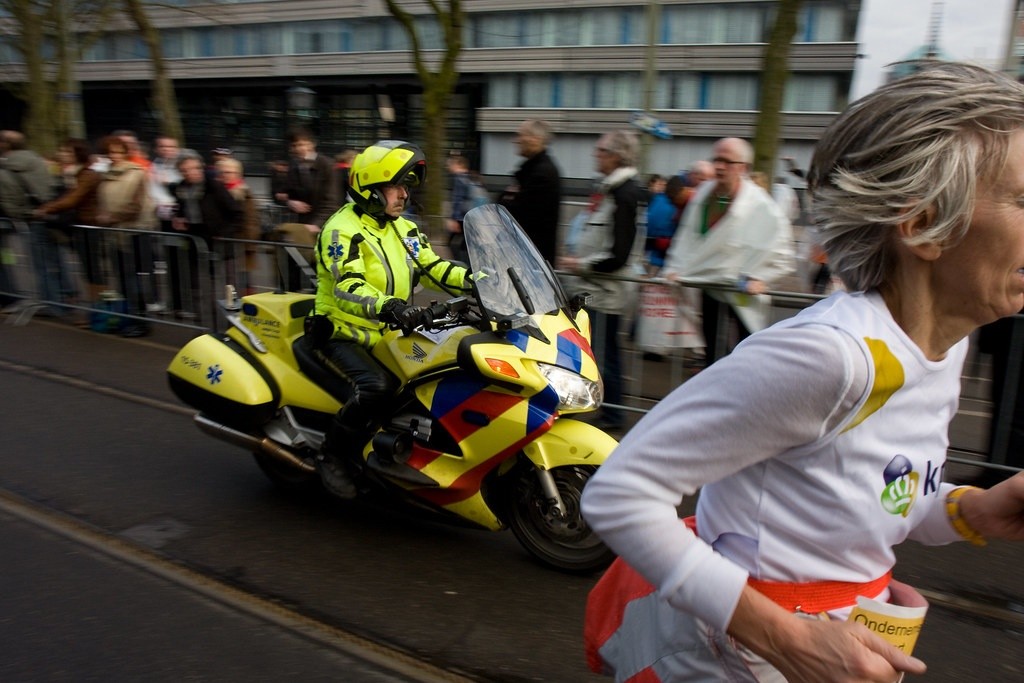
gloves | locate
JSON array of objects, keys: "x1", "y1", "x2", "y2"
[
  {"x1": 378, "y1": 297, "x2": 425, "y2": 336},
  {"x1": 463, "y1": 268, "x2": 496, "y2": 297}
]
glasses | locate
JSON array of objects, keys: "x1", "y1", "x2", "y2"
[{"x1": 712, "y1": 155, "x2": 746, "y2": 167}]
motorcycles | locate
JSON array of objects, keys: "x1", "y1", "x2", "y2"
[{"x1": 167, "y1": 203, "x2": 627, "y2": 576}]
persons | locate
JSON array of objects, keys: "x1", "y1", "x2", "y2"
[
  {"x1": 560, "y1": 128, "x2": 795, "y2": 333},
  {"x1": 0, "y1": 129, "x2": 335, "y2": 335},
  {"x1": 440, "y1": 148, "x2": 496, "y2": 230},
  {"x1": 578, "y1": 55, "x2": 1024, "y2": 683},
  {"x1": 303, "y1": 137, "x2": 473, "y2": 499},
  {"x1": 500, "y1": 119, "x2": 562, "y2": 268}
]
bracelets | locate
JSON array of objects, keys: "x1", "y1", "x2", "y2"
[{"x1": 945, "y1": 486, "x2": 988, "y2": 548}]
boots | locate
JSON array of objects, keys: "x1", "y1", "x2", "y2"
[{"x1": 315, "y1": 409, "x2": 377, "y2": 498}]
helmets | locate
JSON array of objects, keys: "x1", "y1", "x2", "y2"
[{"x1": 348, "y1": 139, "x2": 428, "y2": 216}]
